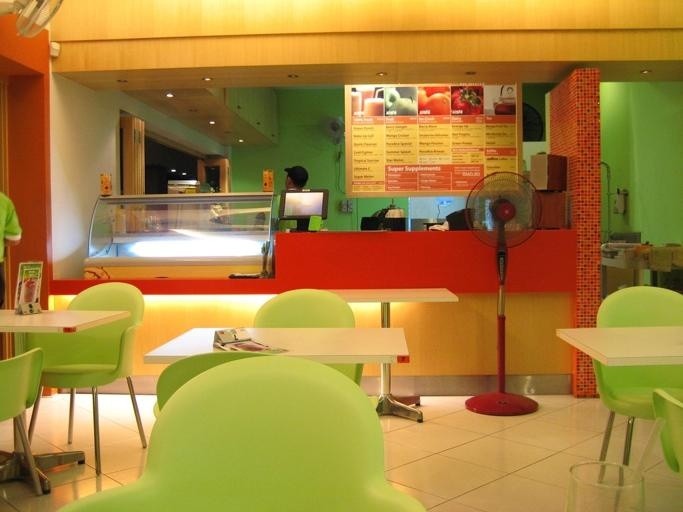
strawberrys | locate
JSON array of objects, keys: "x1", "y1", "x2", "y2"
[{"x1": 451, "y1": 86, "x2": 484, "y2": 115}]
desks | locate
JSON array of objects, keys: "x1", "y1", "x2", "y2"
[
  {"x1": 326, "y1": 288, "x2": 460, "y2": 424},
  {"x1": 553, "y1": 324, "x2": 682, "y2": 369}
]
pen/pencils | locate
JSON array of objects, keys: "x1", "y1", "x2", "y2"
[{"x1": 155, "y1": 277, "x2": 167, "y2": 278}]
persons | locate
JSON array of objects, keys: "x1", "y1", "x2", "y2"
[
  {"x1": 1, "y1": 192, "x2": 23, "y2": 304},
  {"x1": 272, "y1": 164, "x2": 323, "y2": 231}
]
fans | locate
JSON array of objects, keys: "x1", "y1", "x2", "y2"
[
  {"x1": 462, "y1": 172, "x2": 545, "y2": 419},
  {"x1": 0, "y1": 1, "x2": 63, "y2": 38}
]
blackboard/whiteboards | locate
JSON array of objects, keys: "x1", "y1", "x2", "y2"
[{"x1": 409, "y1": 197, "x2": 487, "y2": 233}]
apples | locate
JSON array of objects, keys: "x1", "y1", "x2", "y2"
[
  {"x1": 396, "y1": 96, "x2": 417, "y2": 116},
  {"x1": 384, "y1": 88, "x2": 400, "y2": 111}
]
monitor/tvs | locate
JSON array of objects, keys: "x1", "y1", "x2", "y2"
[{"x1": 279, "y1": 189, "x2": 328, "y2": 232}]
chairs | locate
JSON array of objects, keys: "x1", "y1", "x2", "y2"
[
  {"x1": 0, "y1": 282, "x2": 425, "y2": 510},
  {"x1": 590, "y1": 282, "x2": 682, "y2": 512}
]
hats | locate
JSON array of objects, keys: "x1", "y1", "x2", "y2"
[{"x1": 284, "y1": 166, "x2": 308, "y2": 182}]
